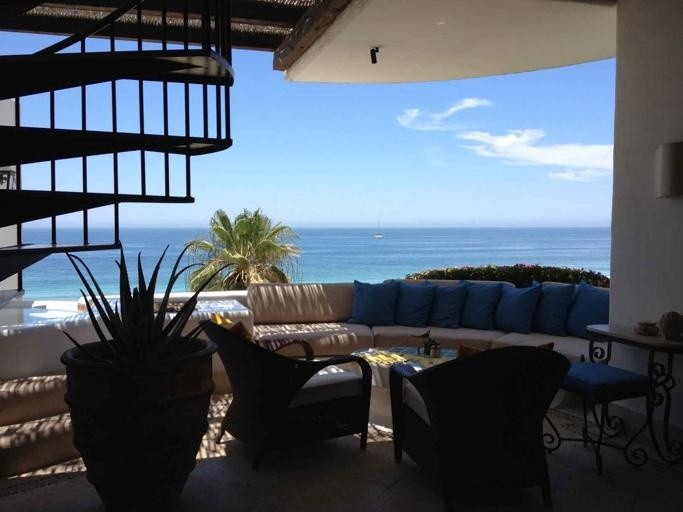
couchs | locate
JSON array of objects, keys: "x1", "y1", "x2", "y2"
[{"x1": 248, "y1": 279, "x2": 609, "y2": 366}]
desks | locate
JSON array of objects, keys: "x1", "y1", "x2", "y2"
[{"x1": 580, "y1": 317, "x2": 679, "y2": 470}]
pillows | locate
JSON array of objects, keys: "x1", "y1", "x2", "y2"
[
  {"x1": 347, "y1": 278, "x2": 608, "y2": 339},
  {"x1": 211, "y1": 312, "x2": 256, "y2": 343}
]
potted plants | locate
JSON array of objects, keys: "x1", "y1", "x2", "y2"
[{"x1": 59, "y1": 236, "x2": 220, "y2": 512}]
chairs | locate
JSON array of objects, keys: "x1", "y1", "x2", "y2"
[
  {"x1": 388, "y1": 340, "x2": 570, "y2": 510},
  {"x1": 199, "y1": 314, "x2": 374, "y2": 474}
]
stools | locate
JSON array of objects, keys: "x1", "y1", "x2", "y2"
[{"x1": 543, "y1": 361, "x2": 654, "y2": 474}]
